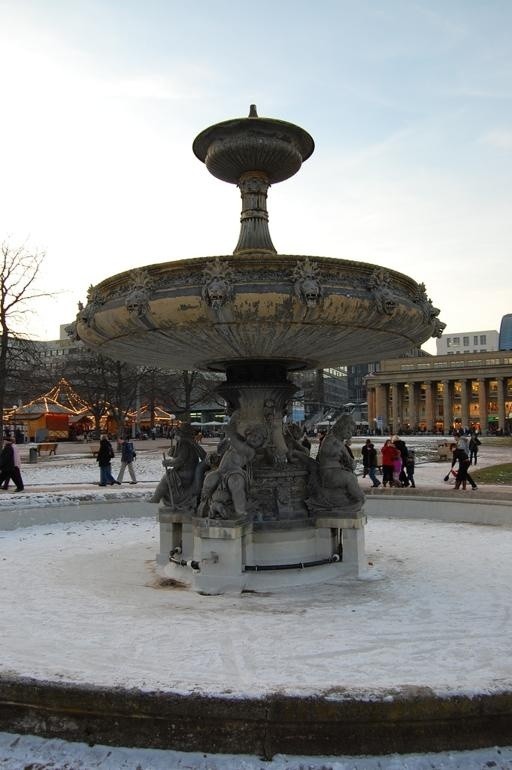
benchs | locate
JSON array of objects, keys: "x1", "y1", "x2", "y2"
[
  {"x1": 377, "y1": 453, "x2": 382, "y2": 473},
  {"x1": 36, "y1": 444, "x2": 58, "y2": 457},
  {"x1": 89, "y1": 446, "x2": 99, "y2": 456}
]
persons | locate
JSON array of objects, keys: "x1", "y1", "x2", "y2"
[
  {"x1": 96, "y1": 434, "x2": 116, "y2": 486},
  {"x1": 116, "y1": 436, "x2": 137, "y2": 485},
  {"x1": 151, "y1": 427, "x2": 155, "y2": 440},
  {"x1": 197, "y1": 423, "x2": 267, "y2": 518},
  {"x1": 316, "y1": 431, "x2": 326, "y2": 461},
  {"x1": 146, "y1": 423, "x2": 199, "y2": 507},
  {"x1": 0, "y1": 436, "x2": 24, "y2": 492},
  {"x1": 361, "y1": 434, "x2": 415, "y2": 487},
  {"x1": 450, "y1": 433, "x2": 481, "y2": 490},
  {"x1": 312, "y1": 414, "x2": 364, "y2": 507},
  {"x1": 301, "y1": 435, "x2": 311, "y2": 450}
]
